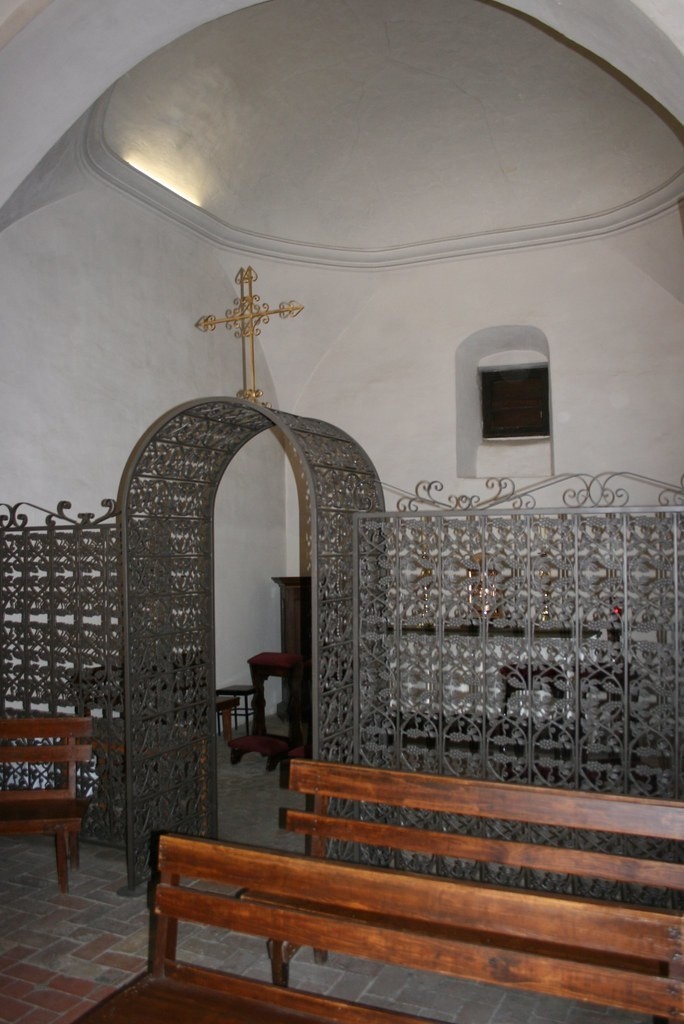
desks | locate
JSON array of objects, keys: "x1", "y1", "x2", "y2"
[{"x1": 248, "y1": 652, "x2": 311, "y2": 736}]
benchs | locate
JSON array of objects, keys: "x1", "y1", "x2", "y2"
[
  {"x1": 0, "y1": 717, "x2": 94, "y2": 894},
  {"x1": 73, "y1": 833, "x2": 684, "y2": 1024},
  {"x1": 240, "y1": 758, "x2": 683, "y2": 989}
]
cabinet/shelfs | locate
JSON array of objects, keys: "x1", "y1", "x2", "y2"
[{"x1": 271, "y1": 576, "x2": 312, "y2": 722}]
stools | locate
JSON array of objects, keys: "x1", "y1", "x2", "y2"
[
  {"x1": 216, "y1": 697, "x2": 240, "y2": 743},
  {"x1": 216, "y1": 685, "x2": 256, "y2": 736},
  {"x1": 229, "y1": 735, "x2": 304, "y2": 772}
]
